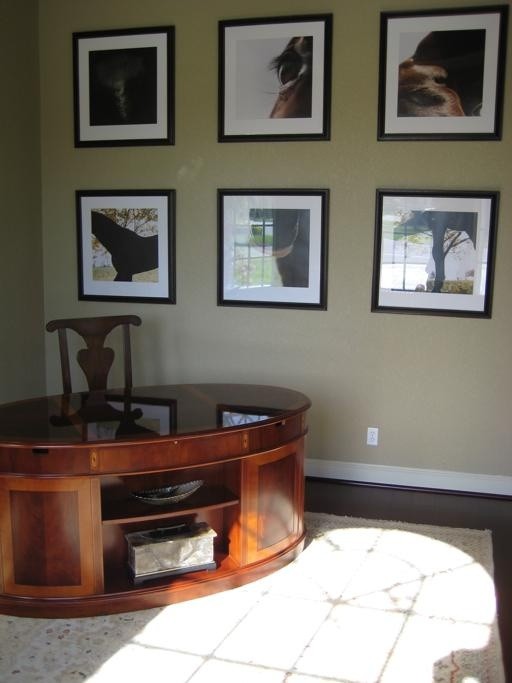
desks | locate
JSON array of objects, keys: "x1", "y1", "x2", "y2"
[{"x1": 0, "y1": 383, "x2": 311, "y2": 619}]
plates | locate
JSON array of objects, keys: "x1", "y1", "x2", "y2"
[{"x1": 130, "y1": 480, "x2": 206, "y2": 505}]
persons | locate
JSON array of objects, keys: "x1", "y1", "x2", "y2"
[{"x1": 266, "y1": 34, "x2": 313, "y2": 119}]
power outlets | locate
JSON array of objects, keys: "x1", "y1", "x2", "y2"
[{"x1": 366, "y1": 427, "x2": 379, "y2": 446}]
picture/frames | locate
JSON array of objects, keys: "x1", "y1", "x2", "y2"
[
  {"x1": 218, "y1": 187, "x2": 330, "y2": 311},
  {"x1": 377, "y1": 3, "x2": 510, "y2": 143},
  {"x1": 370, "y1": 188, "x2": 503, "y2": 318},
  {"x1": 72, "y1": 23, "x2": 176, "y2": 150},
  {"x1": 76, "y1": 187, "x2": 178, "y2": 305},
  {"x1": 216, "y1": 10, "x2": 334, "y2": 143}
]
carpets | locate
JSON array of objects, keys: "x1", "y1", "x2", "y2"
[{"x1": 0, "y1": 511, "x2": 506, "y2": 683}]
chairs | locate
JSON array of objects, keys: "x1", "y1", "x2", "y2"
[{"x1": 45, "y1": 315, "x2": 142, "y2": 393}]
